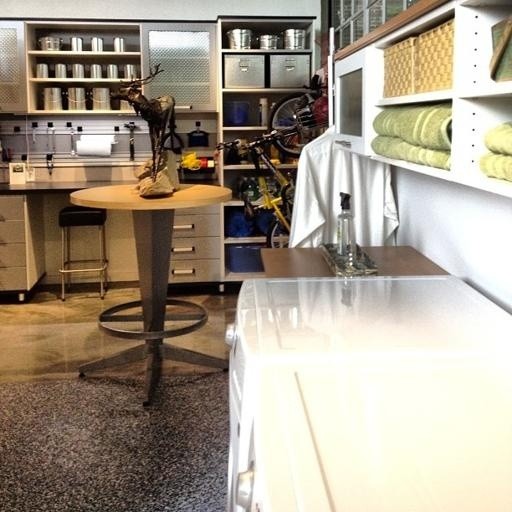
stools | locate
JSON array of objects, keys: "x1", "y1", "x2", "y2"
[{"x1": 57, "y1": 205, "x2": 108, "y2": 302}]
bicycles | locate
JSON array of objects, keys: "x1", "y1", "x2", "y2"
[{"x1": 216, "y1": 93, "x2": 329, "y2": 250}]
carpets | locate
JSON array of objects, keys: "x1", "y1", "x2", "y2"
[{"x1": 0, "y1": 378, "x2": 227, "y2": 512}]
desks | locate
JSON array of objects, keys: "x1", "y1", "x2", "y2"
[
  {"x1": 255, "y1": 243, "x2": 451, "y2": 280},
  {"x1": 68, "y1": 181, "x2": 231, "y2": 407}
]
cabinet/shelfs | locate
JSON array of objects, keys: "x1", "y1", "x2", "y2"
[
  {"x1": 0, "y1": 15, "x2": 218, "y2": 114},
  {"x1": 0, "y1": 146, "x2": 221, "y2": 302},
  {"x1": 217, "y1": 14, "x2": 327, "y2": 292},
  {"x1": 330, "y1": 0, "x2": 512, "y2": 199}
]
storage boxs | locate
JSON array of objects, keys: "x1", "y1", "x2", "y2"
[
  {"x1": 415, "y1": 18, "x2": 461, "y2": 95},
  {"x1": 270, "y1": 54, "x2": 312, "y2": 89},
  {"x1": 223, "y1": 54, "x2": 267, "y2": 89},
  {"x1": 380, "y1": 36, "x2": 416, "y2": 99}
]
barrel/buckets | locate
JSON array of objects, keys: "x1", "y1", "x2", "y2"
[
  {"x1": 259, "y1": 35, "x2": 277, "y2": 50},
  {"x1": 284, "y1": 28, "x2": 306, "y2": 49},
  {"x1": 226, "y1": 29, "x2": 251, "y2": 49}
]
